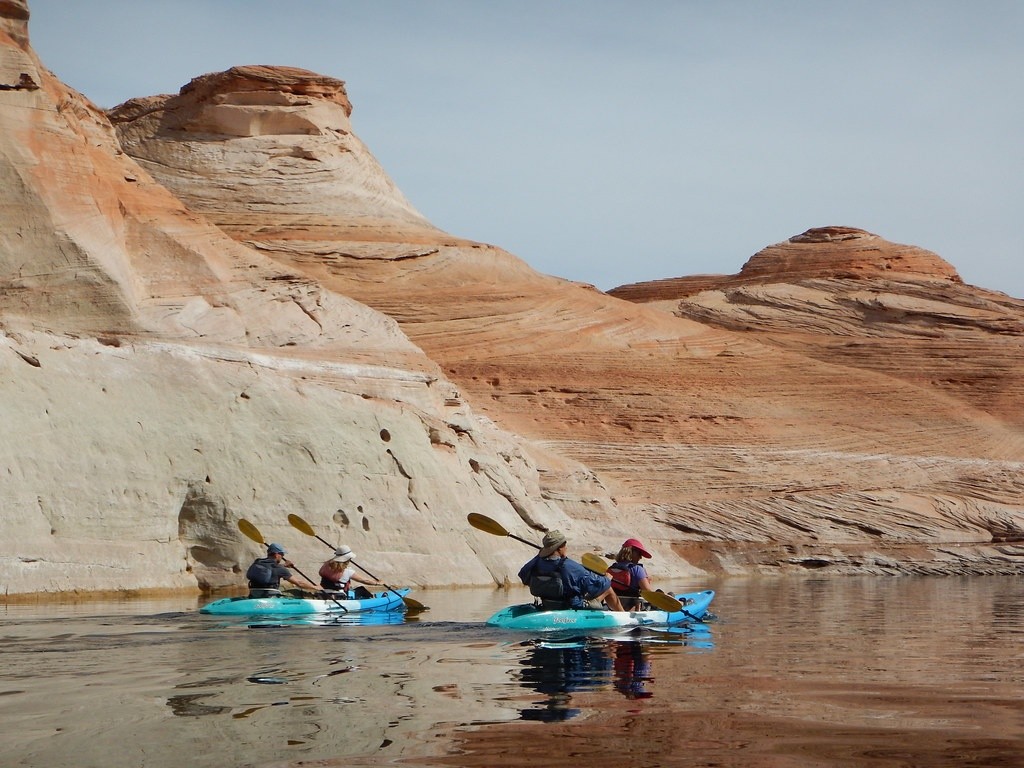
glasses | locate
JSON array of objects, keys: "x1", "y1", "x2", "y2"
[{"x1": 632, "y1": 548, "x2": 643, "y2": 556}]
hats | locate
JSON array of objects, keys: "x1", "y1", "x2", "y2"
[
  {"x1": 623, "y1": 539, "x2": 652, "y2": 559},
  {"x1": 332, "y1": 544, "x2": 357, "y2": 562},
  {"x1": 538, "y1": 529, "x2": 567, "y2": 558},
  {"x1": 266, "y1": 543, "x2": 286, "y2": 554}
]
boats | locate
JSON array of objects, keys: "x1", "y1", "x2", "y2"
[
  {"x1": 198, "y1": 587, "x2": 413, "y2": 616},
  {"x1": 485, "y1": 589, "x2": 716, "y2": 634}
]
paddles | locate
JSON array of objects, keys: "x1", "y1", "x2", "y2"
[
  {"x1": 288, "y1": 514, "x2": 427, "y2": 609},
  {"x1": 466, "y1": 512, "x2": 683, "y2": 613},
  {"x1": 581, "y1": 552, "x2": 704, "y2": 623},
  {"x1": 237, "y1": 518, "x2": 349, "y2": 614}
]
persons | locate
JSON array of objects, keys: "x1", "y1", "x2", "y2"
[
  {"x1": 248, "y1": 543, "x2": 324, "y2": 600},
  {"x1": 318, "y1": 544, "x2": 385, "y2": 600},
  {"x1": 603, "y1": 538, "x2": 688, "y2": 612},
  {"x1": 518, "y1": 530, "x2": 640, "y2": 612}
]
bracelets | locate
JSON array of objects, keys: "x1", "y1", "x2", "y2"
[{"x1": 374, "y1": 581, "x2": 378, "y2": 586}]
